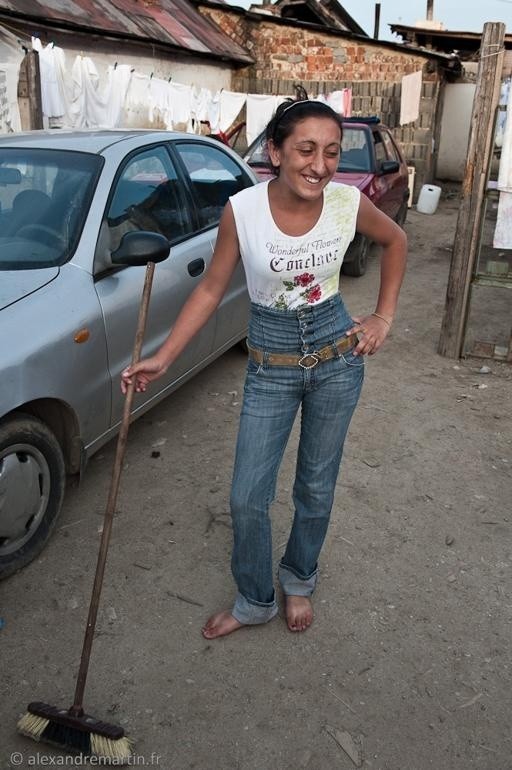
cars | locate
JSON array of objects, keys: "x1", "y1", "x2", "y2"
[
  {"x1": 241, "y1": 120, "x2": 410, "y2": 278},
  {"x1": 0, "y1": 126, "x2": 265, "y2": 583}
]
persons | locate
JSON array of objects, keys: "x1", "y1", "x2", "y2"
[{"x1": 120, "y1": 99, "x2": 410, "y2": 641}]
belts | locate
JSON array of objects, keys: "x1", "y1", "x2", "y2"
[{"x1": 246, "y1": 332, "x2": 359, "y2": 370}]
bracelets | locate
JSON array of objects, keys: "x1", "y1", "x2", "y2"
[{"x1": 371, "y1": 312, "x2": 392, "y2": 328}]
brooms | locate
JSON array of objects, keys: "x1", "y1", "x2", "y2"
[{"x1": 16, "y1": 262, "x2": 157, "y2": 764}]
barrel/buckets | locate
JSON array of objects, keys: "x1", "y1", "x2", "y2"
[{"x1": 416, "y1": 184, "x2": 442, "y2": 215}]
